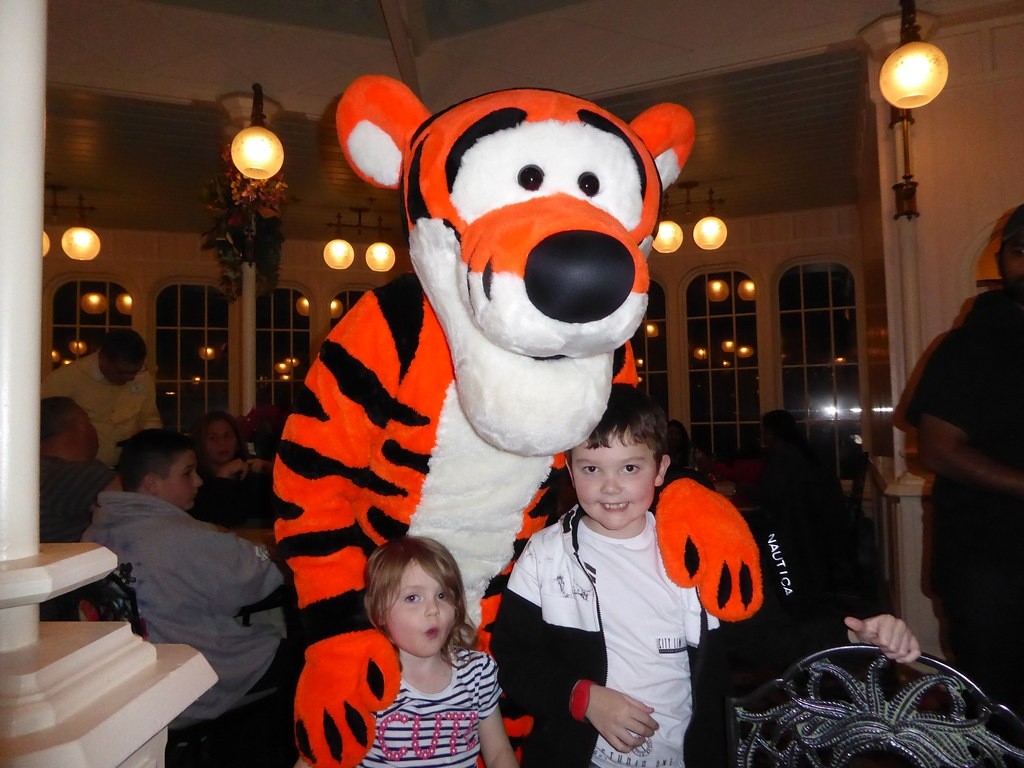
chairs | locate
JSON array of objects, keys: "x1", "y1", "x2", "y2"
[
  {"x1": 723, "y1": 644, "x2": 1024, "y2": 768},
  {"x1": 62, "y1": 562, "x2": 285, "y2": 768}
]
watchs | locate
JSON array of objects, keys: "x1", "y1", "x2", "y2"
[{"x1": 569, "y1": 677, "x2": 599, "y2": 723}]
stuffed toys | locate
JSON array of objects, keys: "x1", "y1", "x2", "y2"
[{"x1": 270, "y1": 69, "x2": 767, "y2": 768}]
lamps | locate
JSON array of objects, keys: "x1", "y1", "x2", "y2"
[
  {"x1": 42, "y1": 82, "x2": 758, "y2": 380},
  {"x1": 879, "y1": 0, "x2": 950, "y2": 222}
]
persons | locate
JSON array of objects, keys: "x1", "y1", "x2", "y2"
[
  {"x1": 287, "y1": 539, "x2": 525, "y2": 768},
  {"x1": 488, "y1": 374, "x2": 922, "y2": 768},
  {"x1": 40, "y1": 323, "x2": 308, "y2": 767},
  {"x1": 655, "y1": 409, "x2": 849, "y2": 613},
  {"x1": 889, "y1": 202, "x2": 1024, "y2": 768}
]
